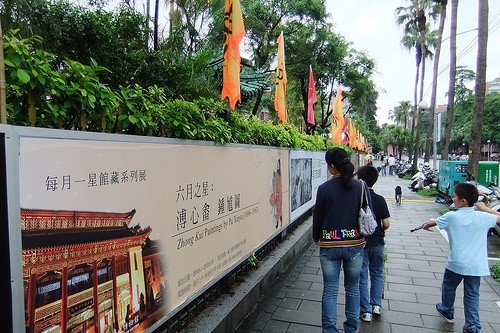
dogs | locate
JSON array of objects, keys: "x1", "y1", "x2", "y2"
[{"x1": 395, "y1": 186, "x2": 402, "y2": 204}]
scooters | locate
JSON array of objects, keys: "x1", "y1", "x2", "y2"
[
  {"x1": 392, "y1": 158, "x2": 413, "y2": 178},
  {"x1": 411, "y1": 165, "x2": 439, "y2": 192},
  {"x1": 449, "y1": 181, "x2": 500, "y2": 238}
]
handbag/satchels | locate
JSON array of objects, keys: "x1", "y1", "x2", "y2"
[{"x1": 358, "y1": 178, "x2": 377, "y2": 236}]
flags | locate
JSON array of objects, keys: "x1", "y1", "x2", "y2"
[
  {"x1": 274, "y1": 30, "x2": 289, "y2": 125},
  {"x1": 332, "y1": 87, "x2": 345, "y2": 144},
  {"x1": 307, "y1": 64, "x2": 318, "y2": 125},
  {"x1": 344, "y1": 114, "x2": 368, "y2": 154},
  {"x1": 221, "y1": 0, "x2": 245, "y2": 111}
]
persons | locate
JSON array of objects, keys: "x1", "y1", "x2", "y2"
[
  {"x1": 312, "y1": 147, "x2": 373, "y2": 333},
  {"x1": 366, "y1": 153, "x2": 396, "y2": 176},
  {"x1": 357, "y1": 165, "x2": 392, "y2": 322},
  {"x1": 423, "y1": 183, "x2": 500, "y2": 333}
]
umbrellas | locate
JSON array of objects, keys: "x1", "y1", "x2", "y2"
[
  {"x1": 410, "y1": 193, "x2": 489, "y2": 236},
  {"x1": 364, "y1": 153, "x2": 375, "y2": 161},
  {"x1": 382, "y1": 232, "x2": 389, "y2": 299}
]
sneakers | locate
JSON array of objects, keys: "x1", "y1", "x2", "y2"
[
  {"x1": 372, "y1": 305, "x2": 381, "y2": 315},
  {"x1": 360, "y1": 312, "x2": 371, "y2": 322},
  {"x1": 436, "y1": 303, "x2": 454, "y2": 324},
  {"x1": 463, "y1": 325, "x2": 479, "y2": 333}
]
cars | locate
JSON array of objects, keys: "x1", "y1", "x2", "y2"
[{"x1": 430, "y1": 153, "x2": 469, "y2": 161}]
